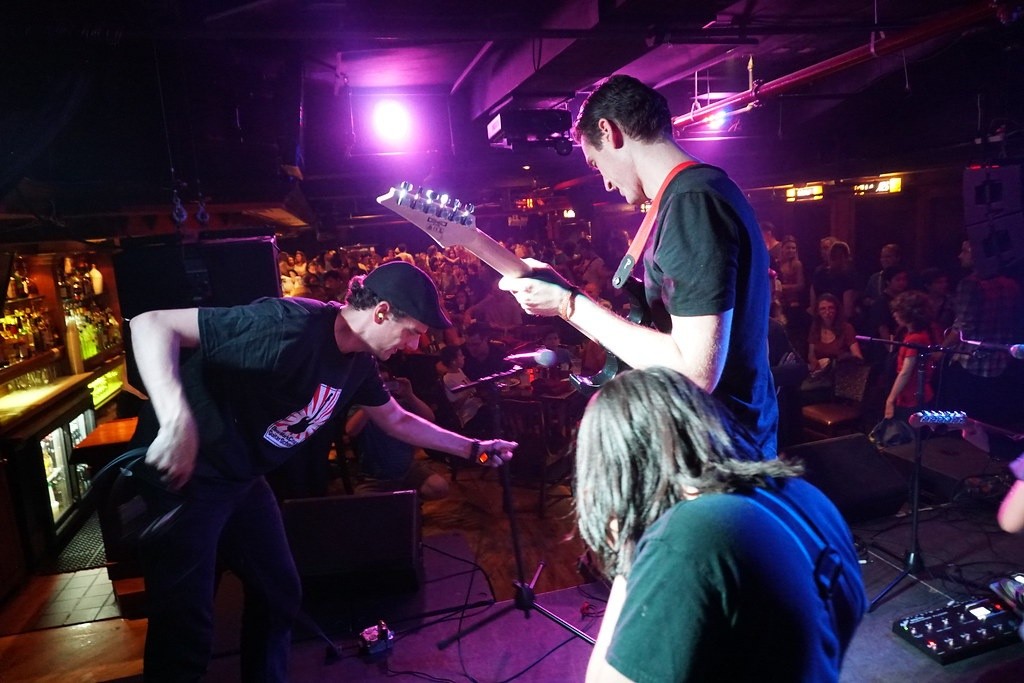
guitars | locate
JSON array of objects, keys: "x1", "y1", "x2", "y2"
[
  {"x1": 908, "y1": 410, "x2": 1023, "y2": 461},
  {"x1": 377, "y1": 181, "x2": 652, "y2": 396}
]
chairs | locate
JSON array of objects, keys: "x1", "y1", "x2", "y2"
[
  {"x1": 799, "y1": 360, "x2": 874, "y2": 439},
  {"x1": 504, "y1": 399, "x2": 574, "y2": 510}
]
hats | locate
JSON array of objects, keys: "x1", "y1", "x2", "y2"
[{"x1": 363, "y1": 261, "x2": 453, "y2": 329}]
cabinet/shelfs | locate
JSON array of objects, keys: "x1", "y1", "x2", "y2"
[{"x1": 0, "y1": 244, "x2": 128, "y2": 560}]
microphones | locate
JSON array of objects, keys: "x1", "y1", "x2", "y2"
[
  {"x1": 961, "y1": 339, "x2": 1024, "y2": 359},
  {"x1": 503, "y1": 348, "x2": 556, "y2": 367}
]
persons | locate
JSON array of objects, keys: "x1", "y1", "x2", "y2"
[
  {"x1": 573, "y1": 365, "x2": 868, "y2": 683},
  {"x1": 129, "y1": 261, "x2": 518, "y2": 683},
  {"x1": 276, "y1": 220, "x2": 1024, "y2": 529},
  {"x1": 497, "y1": 74, "x2": 777, "y2": 466}
]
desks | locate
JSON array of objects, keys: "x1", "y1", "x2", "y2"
[{"x1": 497, "y1": 368, "x2": 598, "y2": 447}]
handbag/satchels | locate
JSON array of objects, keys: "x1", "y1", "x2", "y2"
[{"x1": 868, "y1": 418, "x2": 918, "y2": 456}]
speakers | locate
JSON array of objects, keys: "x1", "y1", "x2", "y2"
[
  {"x1": 780, "y1": 432, "x2": 909, "y2": 527},
  {"x1": 280, "y1": 487, "x2": 425, "y2": 602},
  {"x1": 112, "y1": 234, "x2": 282, "y2": 397},
  {"x1": 957, "y1": 165, "x2": 1024, "y2": 461}
]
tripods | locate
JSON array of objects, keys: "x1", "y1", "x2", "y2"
[
  {"x1": 853, "y1": 335, "x2": 994, "y2": 614},
  {"x1": 436, "y1": 365, "x2": 596, "y2": 650}
]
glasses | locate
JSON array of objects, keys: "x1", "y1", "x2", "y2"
[
  {"x1": 818, "y1": 306, "x2": 837, "y2": 313},
  {"x1": 466, "y1": 340, "x2": 483, "y2": 348}
]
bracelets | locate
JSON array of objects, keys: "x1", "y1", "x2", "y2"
[
  {"x1": 467, "y1": 439, "x2": 479, "y2": 464},
  {"x1": 562, "y1": 287, "x2": 578, "y2": 322},
  {"x1": 611, "y1": 568, "x2": 628, "y2": 578}
]
